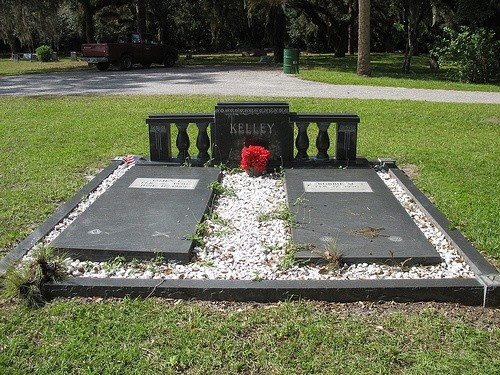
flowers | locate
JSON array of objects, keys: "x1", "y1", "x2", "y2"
[{"x1": 240, "y1": 144, "x2": 271, "y2": 173}]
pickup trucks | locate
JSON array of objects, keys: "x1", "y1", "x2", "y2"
[{"x1": 80, "y1": 31, "x2": 179, "y2": 71}]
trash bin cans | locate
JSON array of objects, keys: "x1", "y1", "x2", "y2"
[{"x1": 283, "y1": 48, "x2": 299, "y2": 74}]
